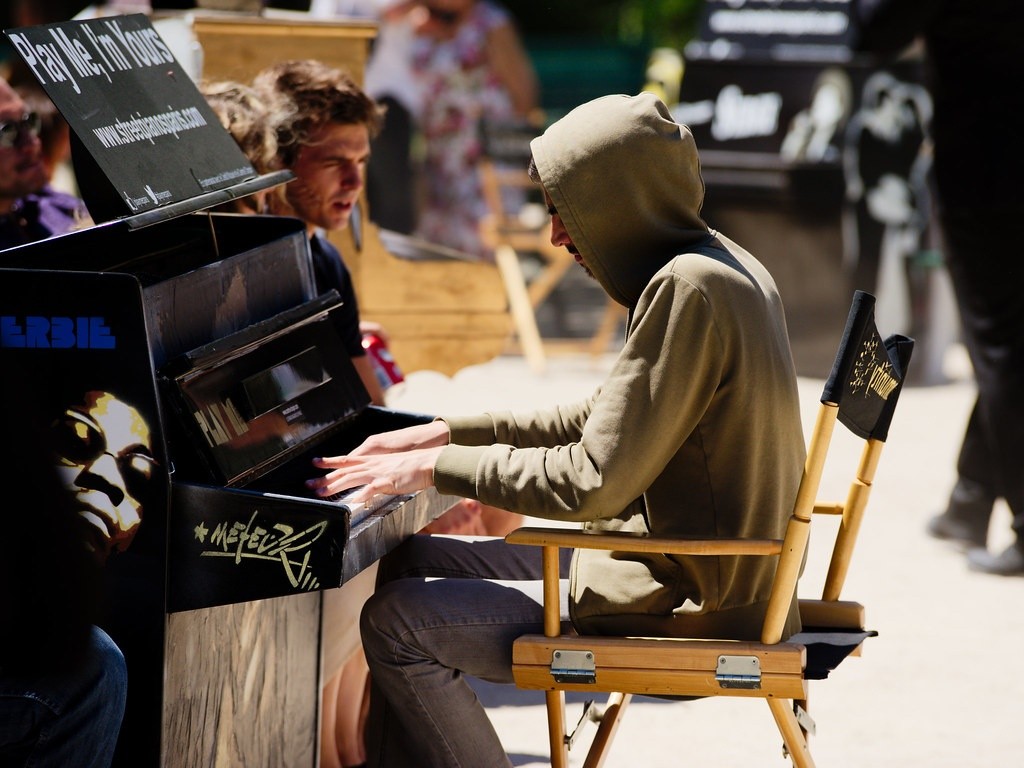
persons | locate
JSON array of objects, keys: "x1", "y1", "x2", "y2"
[
  {"x1": 305, "y1": 91, "x2": 811, "y2": 768},
  {"x1": 362, "y1": 0, "x2": 545, "y2": 263},
  {"x1": 780, "y1": 0, "x2": 1024, "y2": 577},
  {"x1": 0, "y1": 62, "x2": 95, "y2": 249},
  {"x1": 201, "y1": 59, "x2": 388, "y2": 406},
  {"x1": 70, "y1": 0, "x2": 154, "y2": 21},
  {"x1": 0, "y1": 389, "x2": 160, "y2": 768}
]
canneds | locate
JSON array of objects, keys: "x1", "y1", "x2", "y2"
[{"x1": 362, "y1": 333, "x2": 405, "y2": 388}]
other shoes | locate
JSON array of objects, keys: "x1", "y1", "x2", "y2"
[
  {"x1": 928, "y1": 512, "x2": 993, "y2": 550},
  {"x1": 968, "y1": 544, "x2": 1024, "y2": 577}
]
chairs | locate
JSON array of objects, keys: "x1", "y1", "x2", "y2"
[{"x1": 504, "y1": 291, "x2": 917, "y2": 766}]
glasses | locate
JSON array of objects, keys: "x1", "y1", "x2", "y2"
[
  {"x1": 32, "y1": 408, "x2": 166, "y2": 494},
  {"x1": 0, "y1": 111, "x2": 42, "y2": 147}
]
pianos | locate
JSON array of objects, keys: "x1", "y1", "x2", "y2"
[
  {"x1": 149, "y1": 7, "x2": 514, "y2": 379},
  {"x1": 0, "y1": 202, "x2": 469, "y2": 767},
  {"x1": 674, "y1": 37, "x2": 949, "y2": 391}
]
